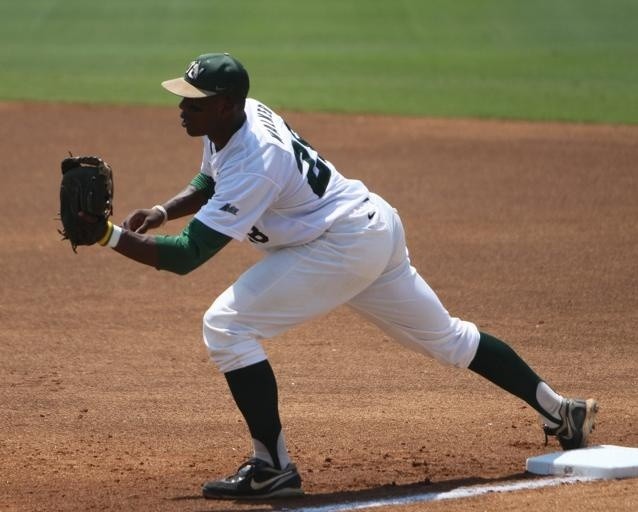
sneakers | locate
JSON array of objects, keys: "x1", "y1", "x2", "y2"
[
  {"x1": 202, "y1": 459, "x2": 304, "y2": 501},
  {"x1": 544, "y1": 398, "x2": 598, "y2": 451}
]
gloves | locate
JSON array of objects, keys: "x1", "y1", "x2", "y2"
[{"x1": 63, "y1": 215, "x2": 122, "y2": 250}]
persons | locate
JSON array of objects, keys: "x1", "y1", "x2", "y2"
[{"x1": 60, "y1": 53, "x2": 599, "y2": 500}]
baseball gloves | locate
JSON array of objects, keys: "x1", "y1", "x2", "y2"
[{"x1": 54, "y1": 151, "x2": 113, "y2": 253}]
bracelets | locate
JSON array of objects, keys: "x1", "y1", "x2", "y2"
[{"x1": 152, "y1": 205, "x2": 167, "y2": 227}]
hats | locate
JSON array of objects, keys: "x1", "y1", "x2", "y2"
[{"x1": 161, "y1": 53, "x2": 249, "y2": 100}]
